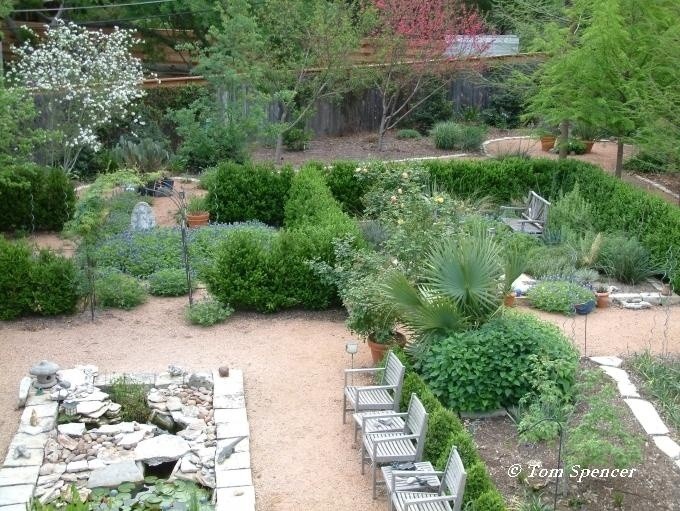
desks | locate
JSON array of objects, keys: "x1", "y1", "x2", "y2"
[
  {"x1": 380, "y1": 461, "x2": 441, "y2": 493},
  {"x1": 352, "y1": 410, "x2": 405, "y2": 443}
]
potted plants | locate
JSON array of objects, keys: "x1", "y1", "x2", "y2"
[
  {"x1": 504, "y1": 241, "x2": 533, "y2": 306},
  {"x1": 537, "y1": 126, "x2": 561, "y2": 151},
  {"x1": 579, "y1": 121, "x2": 597, "y2": 153},
  {"x1": 594, "y1": 280, "x2": 610, "y2": 308},
  {"x1": 115, "y1": 169, "x2": 174, "y2": 197},
  {"x1": 186, "y1": 194, "x2": 212, "y2": 226},
  {"x1": 527, "y1": 281, "x2": 594, "y2": 315},
  {"x1": 301, "y1": 232, "x2": 406, "y2": 363}
]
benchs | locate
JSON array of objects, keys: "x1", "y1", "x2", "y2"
[{"x1": 499, "y1": 190, "x2": 551, "y2": 235}]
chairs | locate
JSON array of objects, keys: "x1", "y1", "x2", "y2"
[
  {"x1": 391, "y1": 445, "x2": 468, "y2": 511},
  {"x1": 361, "y1": 392, "x2": 430, "y2": 500},
  {"x1": 341, "y1": 349, "x2": 406, "y2": 443}
]
